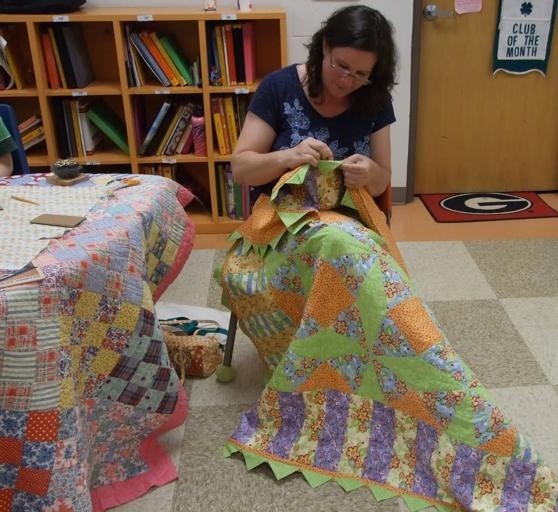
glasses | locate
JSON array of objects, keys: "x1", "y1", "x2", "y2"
[{"x1": 329, "y1": 48, "x2": 369, "y2": 86}]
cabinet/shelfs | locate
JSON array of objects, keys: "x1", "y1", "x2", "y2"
[{"x1": 0, "y1": 7, "x2": 287, "y2": 234}]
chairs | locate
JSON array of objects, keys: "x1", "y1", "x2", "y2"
[
  {"x1": 0, "y1": 104, "x2": 30, "y2": 176},
  {"x1": 216, "y1": 180, "x2": 391, "y2": 381}
]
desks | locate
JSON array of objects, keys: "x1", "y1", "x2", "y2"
[{"x1": 0, "y1": 172, "x2": 198, "y2": 512}]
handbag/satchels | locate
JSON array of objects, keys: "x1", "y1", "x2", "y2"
[{"x1": 155, "y1": 318, "x2": 228, "y2": 377}]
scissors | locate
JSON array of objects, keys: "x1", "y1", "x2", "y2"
[
  {"x1": 109, "y1": 179, "x2": 142, "y2": 193},
  {"x1": 107, "y1": 173, "x2": 127, "y2": 185}
]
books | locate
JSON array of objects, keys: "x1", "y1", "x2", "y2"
[
  {"x1": 126, "y1": 24, "x2": 253, "y2": 223},
  {"x1": 0, "y1": 23, "x2": 124, "y2": 156}
]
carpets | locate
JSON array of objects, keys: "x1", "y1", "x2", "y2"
[
  {"x1": 100, "y1": 238, "x2": 558, "y2": 512},
  {"x1": 419, "y1": 191, "x2": 558, "y2": 223}
]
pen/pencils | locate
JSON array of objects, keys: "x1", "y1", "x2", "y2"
[{"x1": 10, "y1": 194, "x2": 41, "y2": 206}]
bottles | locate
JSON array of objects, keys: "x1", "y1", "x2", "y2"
[{"x1": 190, "y1": 105, "x2": 207, "y2": 157}]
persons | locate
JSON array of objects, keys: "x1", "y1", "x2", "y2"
[{"x1": 232, "y1": 6, "x2": 396, "y2": 204}]
